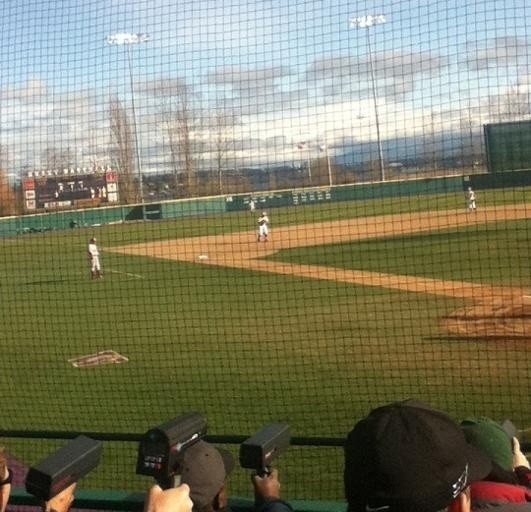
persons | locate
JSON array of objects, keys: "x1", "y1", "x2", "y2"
[
  {"x1": 89, "y1": 185, "x2": 106, "y2": 199},
  {"x1": 54, "y1": 190, "x2": 59, "y2": 199},
  {"x1": 256, "y1": 211, "x2": 270, "y2": 242},
  {"x1": 0, "y1": 399, "x2": 530, "y2": 511},
  {"x1": 468, "y1": 187, "x2": 476, "y2": 213},
  {"x1": 88, "y1": 237, "x2": 103, "y2": 280},
  {"x1": 249, "y1": 198, "x2": 256, "y2": 216}
]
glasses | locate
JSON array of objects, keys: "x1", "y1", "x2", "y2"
[{"x1": 0, "y1": 468, "x2": 17, "y2": 487}]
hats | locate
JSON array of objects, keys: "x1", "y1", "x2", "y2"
[
  {"x1": 342, "y1": 398, "x2": 493, "y2": 512},
  {"x1": 171, "y1": 438, "x2": 236, "y2": 509},
  {"x1": 459, "y1": 416, "x2": 514, "y2": 472}
]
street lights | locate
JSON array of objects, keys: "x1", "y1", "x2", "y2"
[
  {"x1": 102, "y1": 30, "x2": 158, "y2": 220},
  {"x1": 344, "y1": 13, "x2": 393, "y2": 187}
]
